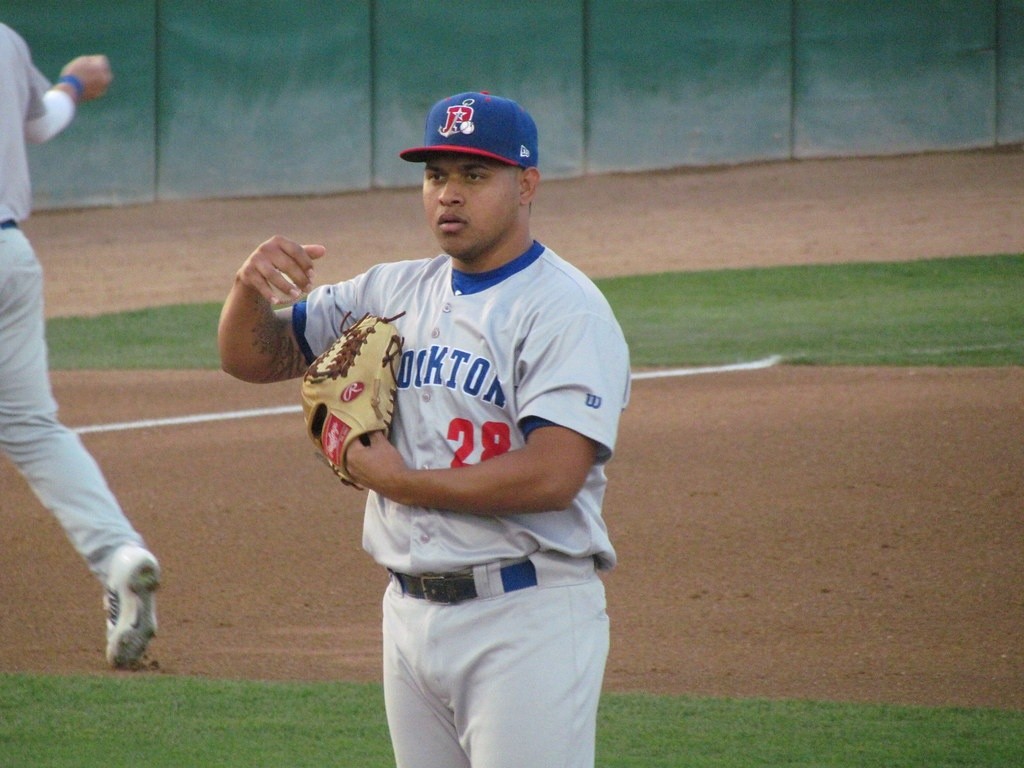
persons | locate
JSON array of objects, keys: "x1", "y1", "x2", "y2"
[
  {"x1": 218, "y1": 90, "x2": 632, "y2": 768},
  {"x1": 0, "y1": 23, "x2": 163, "y2": 667}
]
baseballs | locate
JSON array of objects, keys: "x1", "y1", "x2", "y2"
[{"x1": 267, "y1": 268, "x2": 303, "y2": 305}]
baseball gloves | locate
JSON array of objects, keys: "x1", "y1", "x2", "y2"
[{"x1": 299, "y1": 309, "x2": 408, "y2": 494}]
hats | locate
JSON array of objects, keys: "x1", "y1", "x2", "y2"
[{"x1": 400, "y1": 91, "x2": 539, "y2": 167}]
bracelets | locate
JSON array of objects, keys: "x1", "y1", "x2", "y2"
[{"x1": 56, "y1": 75, "x2": 84, "y2": 97}]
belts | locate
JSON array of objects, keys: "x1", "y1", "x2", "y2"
[
  {"x1": 1, "y1": 219, "x2": 18, "y2": 230},
  {"x1": 387, "y1": 560, "x2": 537, "y2": 604}
]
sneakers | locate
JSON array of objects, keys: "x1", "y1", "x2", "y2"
[{"x1": 103, "y1": 545, "x2": 161, "y2": 666}]
